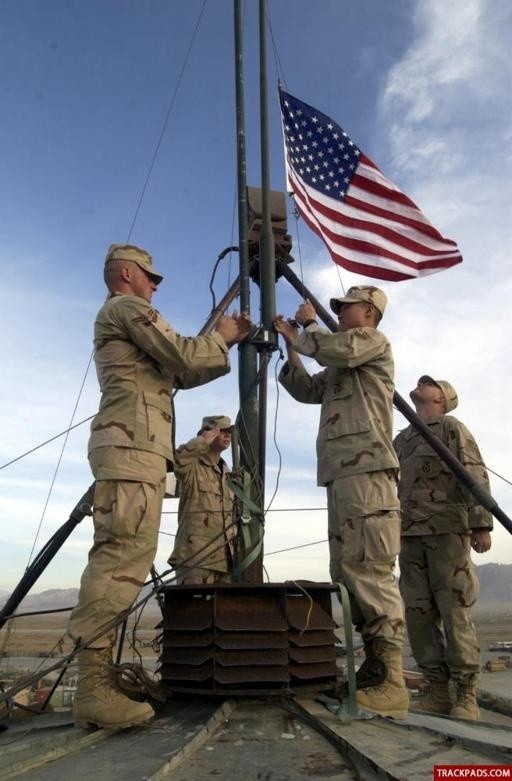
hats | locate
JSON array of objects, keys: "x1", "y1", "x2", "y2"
[
  {"x1": 330, "y1": 286, "x2": 388, "y2": 319},
  {"x1": 104, "y1": 243, "x2": 164, "y2": 285},
  {"x1": 418, "y1": 376, "x2": 458, "y2": 414},
  {"x1": 341, "y1": 640, "x2": 410, "y2": 720},
  {"x1": 203, "y1": 416, "x2": 235, "y2": 433}
]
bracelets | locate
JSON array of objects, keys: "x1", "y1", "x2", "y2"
[{"x1": 302, "y1": 319, "x2": 318, "y2": 331}]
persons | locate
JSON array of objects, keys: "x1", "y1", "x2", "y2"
[
  {"x1": 391, "y1": 374, "x2": 494, "y2": 725},
  {"x1": 68, "y1": 242, "x2": 253, "y2": 733},
  {"x1": 270, "y1": 283, "x2": 412, "y2": 721},
  {"x1": 171, "y1": 414, "x2": 240, "y2": 588}
]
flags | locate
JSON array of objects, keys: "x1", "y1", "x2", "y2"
[{"x1": 277, "y1": 77, "x2": 463, "y2": 284}]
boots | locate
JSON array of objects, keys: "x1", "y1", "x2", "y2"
[
  {"x1": 449, "y1": 682, "x2": 482, "y2": 721},
  {"x1": 409, "y1": 682, "x2": 453, "y2": 715},
  {"x1": 72, "y1": 647, "x2": 156, "y2": 731}
]
